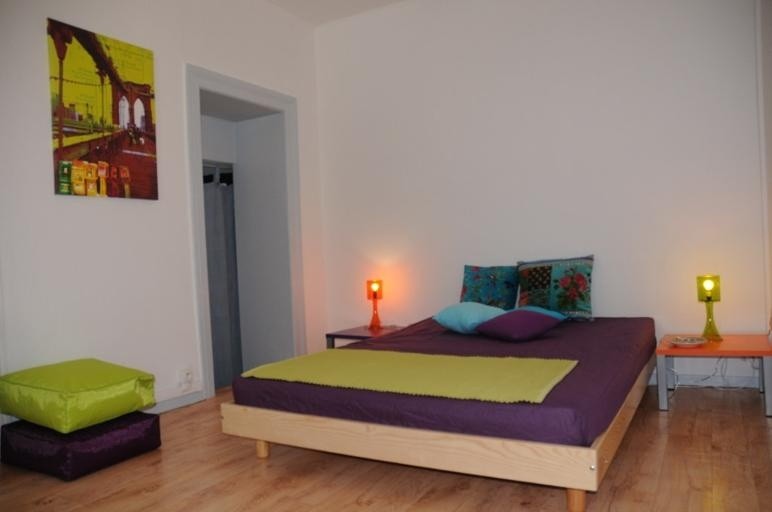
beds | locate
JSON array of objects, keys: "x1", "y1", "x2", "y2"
[{"x1": 216, "y1": 308, "x2": 659, "y2": 509}]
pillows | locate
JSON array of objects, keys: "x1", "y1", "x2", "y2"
[
  {"x1": 517, "y1": 253, "x2": 597, "y2": 319},
  {"x1": 459, "y1": 262, "x2": 519, "y2": 309},
  {"x1": 432, "y1": 299, "x2": 506, "y2": 337},
  {"x1": 474, "y1": 305, "x2": 569, "y2": 343}
]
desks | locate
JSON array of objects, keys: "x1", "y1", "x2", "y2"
[
  {"x1": 654, "y1": 332, "x2": 772, "y2": 421},
  {"x1": 322, "y1": 323, "x2": 406, "y2": 348}
]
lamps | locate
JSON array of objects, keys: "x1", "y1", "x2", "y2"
[
  {"x1": 691, "y1": 272, "x2": 726, "y2": 342},
  {"x1": 365, "y1": 278, "x2": 385, "y2": 330}
]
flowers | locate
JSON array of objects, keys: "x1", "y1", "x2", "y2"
[{"x1": 550, "y1": 264, "x2": 591, "y2": 312}]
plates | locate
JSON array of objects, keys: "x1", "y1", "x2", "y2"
[{"x1": 669, "y1": 334, "x2": 708, "y2": 349}]
